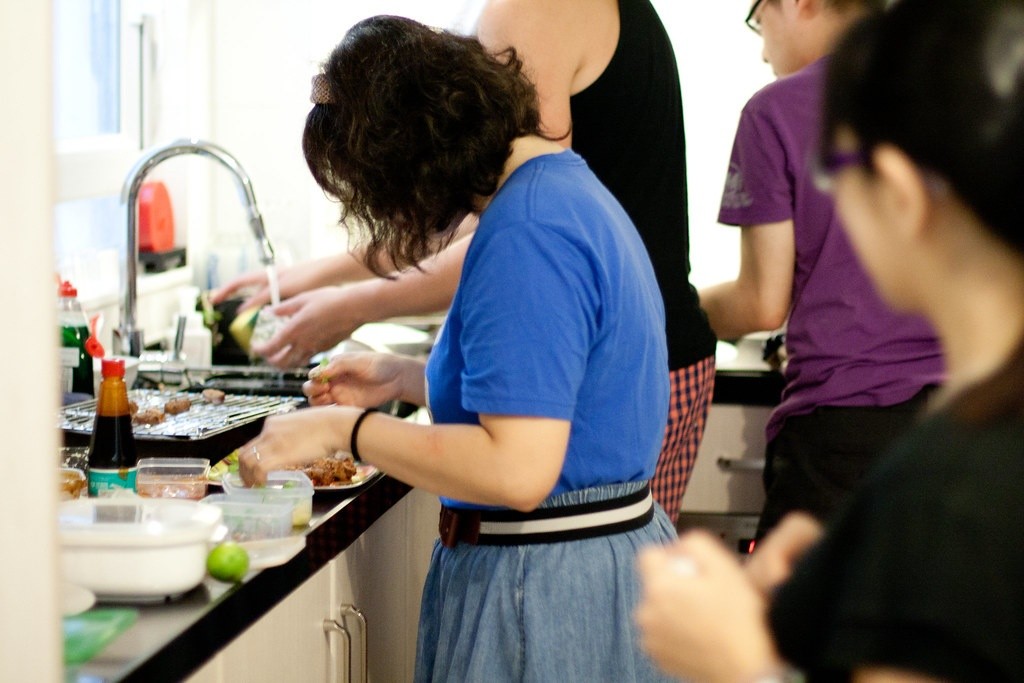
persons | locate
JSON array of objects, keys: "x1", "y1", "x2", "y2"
[
  {"x1": 237, "y1": 16, "x2": 678, "y2": 683},
  {"x1": 631, "y1": 0, "x2": 1023, "y2": 683},
  {"x1": 700, "y1": 0, "x2": 947, "y2": 683},
  {"x1": 209, "y1": 0, "x2": 718, "y2": 524}
]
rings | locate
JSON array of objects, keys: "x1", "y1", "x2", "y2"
[{"x1": 252, "y1": 447, "x2": 261, "y2": 460}]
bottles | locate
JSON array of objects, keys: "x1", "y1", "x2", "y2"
[
  {"x1": 58, "y1": 280, "x2": 93, "y2": 398},
  {"x1": 88, "y1": 357, "x2": 138, "y2": 492}
]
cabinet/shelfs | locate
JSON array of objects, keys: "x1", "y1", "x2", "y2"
[{"x1": 176, "y1": 403, "x2": 785, "y2": 683}]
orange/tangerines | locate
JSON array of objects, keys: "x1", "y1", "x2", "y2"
[{"x1": 208, "y1": 544, "x2": 248, "y2": 582}]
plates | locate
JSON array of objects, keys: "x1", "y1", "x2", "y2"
[{"x1": 206, "y1": 444, "x2": 377, "y2": 489}]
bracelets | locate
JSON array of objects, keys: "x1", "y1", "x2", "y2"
[
  {"x1": 756, "y1": 666, "x2": 805, "y2": 683},
  {"x1": 350, "y1": 407, "x2": 379, "y2": 462}
]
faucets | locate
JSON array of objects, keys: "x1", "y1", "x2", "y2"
[{"x1": 110, "y1": 138, "x2": 275, "y2": 359}]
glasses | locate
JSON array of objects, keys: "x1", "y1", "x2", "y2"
[
  {"x1": 810, "y1": 154, "x2": 860, "y2": 196},
  {"x1": 744, "y1": 1, "x2": 766, "y2": 33}
]
